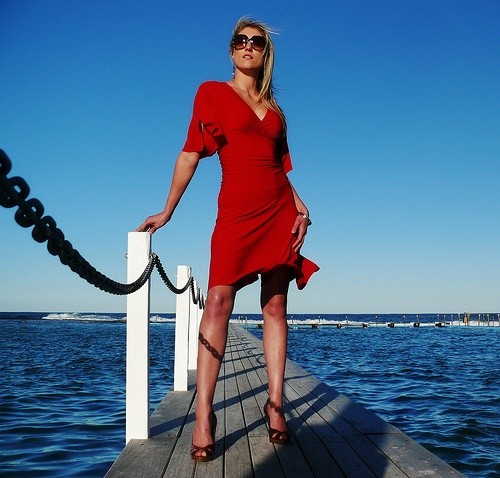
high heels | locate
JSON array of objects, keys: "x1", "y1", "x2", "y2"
[
  {"x1": 191, "y1": 412, "x2": 218, "y2": 462},
  {"x1": 263, "y1": 398, "x2": 292, "y2": 445}
]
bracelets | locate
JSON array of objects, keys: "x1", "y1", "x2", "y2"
[{"x1": 297, "y1": 212, "x2": 313, "y2": 227}]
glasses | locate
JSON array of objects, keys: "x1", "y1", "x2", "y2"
[{"x1": 232, "y1": 34, "x2": 267, "y2": 52}]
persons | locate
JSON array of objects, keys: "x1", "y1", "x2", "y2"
[{"x1": 134, "y1": 16, "x2": 321, "y2": 463}]
